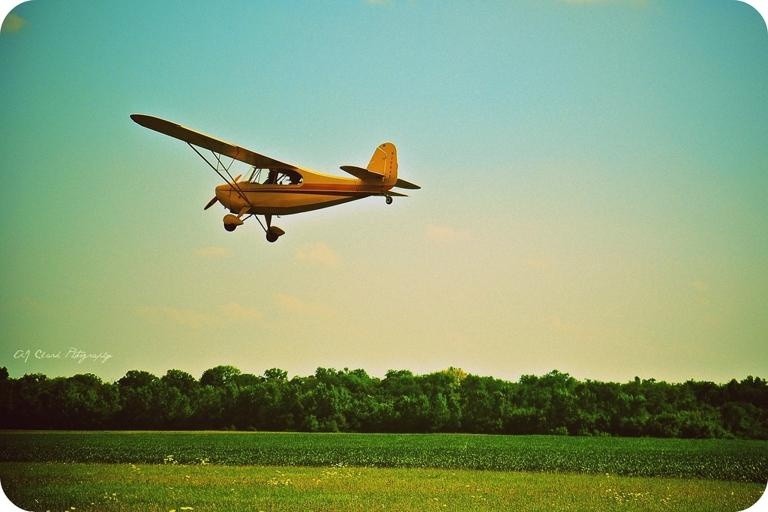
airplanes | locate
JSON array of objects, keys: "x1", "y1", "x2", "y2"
[{"x1": 128, "y1": 111, "x2": 423, "y2": 245}]
